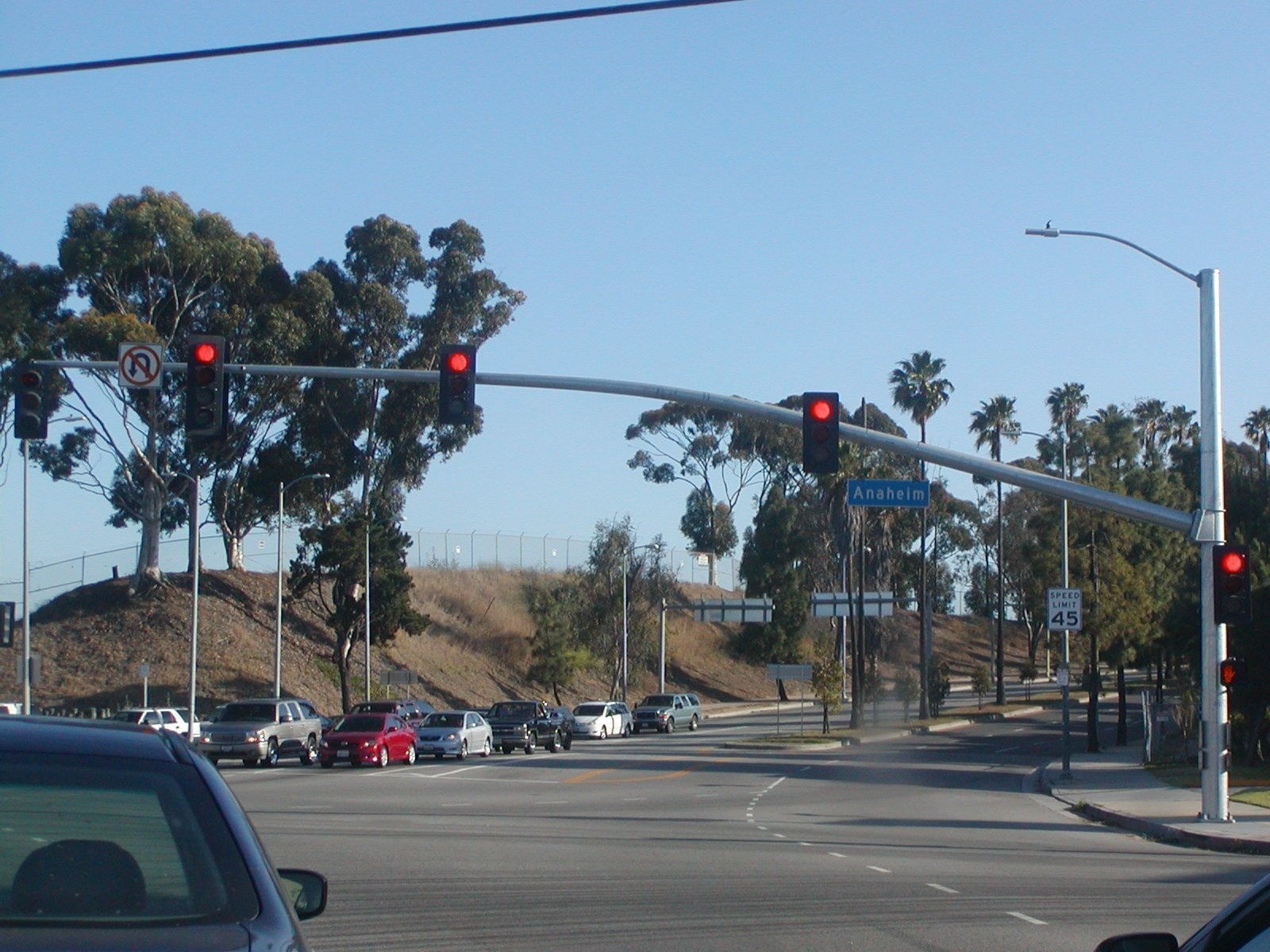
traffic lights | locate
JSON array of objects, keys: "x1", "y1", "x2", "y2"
[
  {"x1": 803, "y1": 392, "x2": 839, "y2": 475},
  {"x1": 184, "y1": 333, "x2": 225, "y2": 442},
  {"x1": 161, "y1": 471, "x2": 201, "y2": 742},
  {"x1": 1212, "y1": 544, "x2": 1251, "y2": 626},
  {"x1": 14, "y1": 361, "x2": 47, "y2": 440},
  {"x1": 1220, "y1": 659, "x2": 1242, "y2": 687},
  {"x1": 438, "y1": 345, "x2": 476, "y2": 426}
]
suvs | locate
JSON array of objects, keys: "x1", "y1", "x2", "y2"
[
  {"x1": 631, "y1": 692, "x2": 702, "y2": 734},
  {"x1": 197, "y1": 698, "x2": 322, "y2": 769}
]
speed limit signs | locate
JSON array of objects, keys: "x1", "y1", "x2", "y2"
[{"x1": 1046, "y1": 589, "x2": 1083, "y2": 632}]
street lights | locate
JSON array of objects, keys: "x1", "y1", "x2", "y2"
[
  {"x1": 841, "y1": 545, "x2": 872, "y2": 699},
  {"x1": 272, "y1": 473, "x2": 330, "y2": 697},
  {"x1": 623, "y1": 543, "x2": 659, "y2": 701},
  {"x1": 1026, "y1": 220, "x2": 1230, "y2": 824},
  {"x1": 1001, "y1": 429, "x2": 1072, "y2": 780},
  {"x1": 366, "y1": 517, "x2": 405, "y2": 709}
]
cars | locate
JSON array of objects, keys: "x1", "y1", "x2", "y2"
[
  {"x1": 0, "y1": 713, "x2": 328, "y2": 952},
  {"x1": 470, "y1": 700, "x2": 575, "y2": 755},
  {"x1": 570, "y1": 701, "x2": 634, "y2": 739},
  {"x1": 107, "y1": 707, "x2": 201, "y2": 743},
  {"x1": 297, "y1": 698, "x2": 436, "y2": 768},
  {"x1": 412, "y1": 709, "x2": 494, "y2": 761}
]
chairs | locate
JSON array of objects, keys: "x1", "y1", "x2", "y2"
[
  {"x1": 495, "y1": 705, "x2": 511, "y2": 716},
  {"x1": 6, "y1": 835, "x2": 149, "y2": 919}
]
